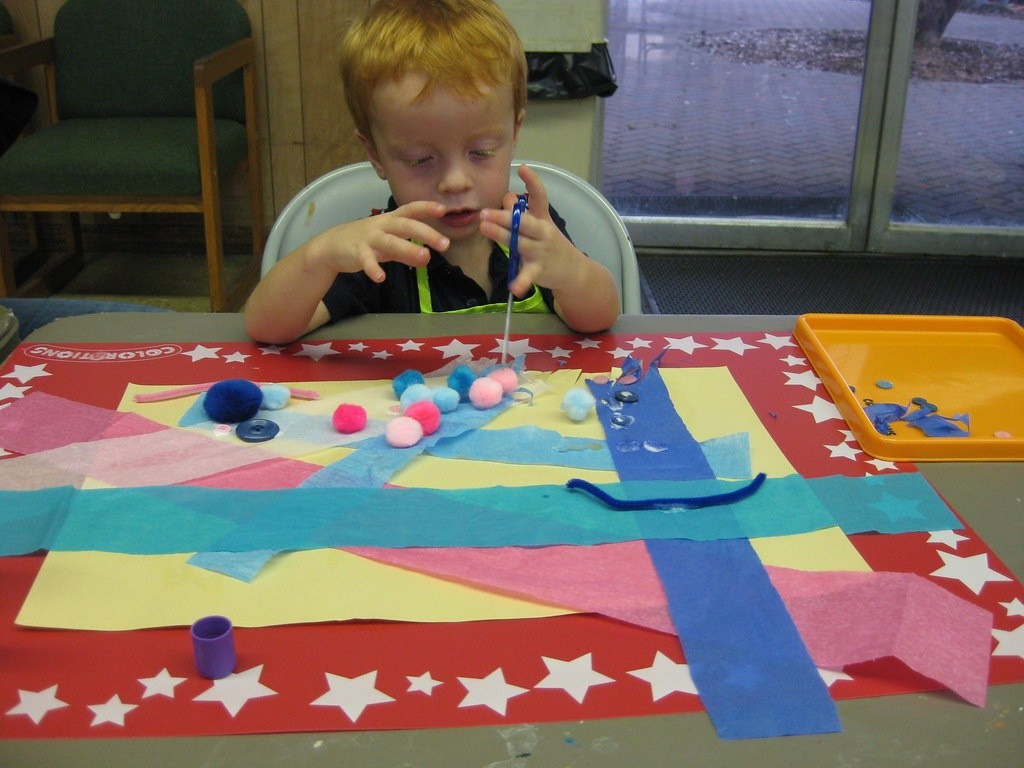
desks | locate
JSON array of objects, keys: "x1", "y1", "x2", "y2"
[{"x1": 0, "y1": 310, "x2": 1024, "y2": 768}]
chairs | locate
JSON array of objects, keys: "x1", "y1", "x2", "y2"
[
  {"x1": 0, "y1": 0, "x2": 263, "y2": 311},
  {"x1": 257, "y1": 161, "x2": 643, "y2": 316}
]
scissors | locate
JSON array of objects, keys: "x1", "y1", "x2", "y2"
[{"x1": 501, "y1": 192, "x2": 528, "y2": 363}]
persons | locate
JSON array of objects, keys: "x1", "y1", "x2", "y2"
[{"x1": 244, "y1": 0, "x2": 620, "y2": 345}]
